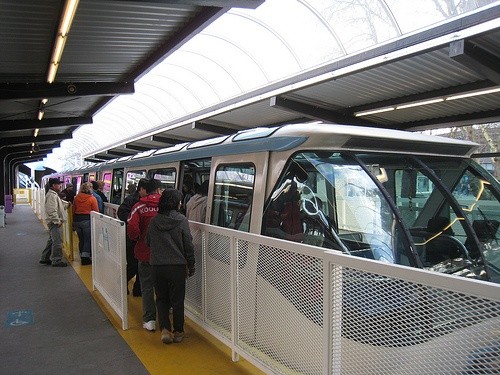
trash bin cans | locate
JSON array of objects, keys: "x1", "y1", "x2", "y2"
[{"x1": 5, "y1": 195, "x2": 12, "y2": 213}]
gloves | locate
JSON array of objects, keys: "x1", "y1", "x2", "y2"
[{"x1": 188, "y1": 267, "x2": 195, "y2": 277}]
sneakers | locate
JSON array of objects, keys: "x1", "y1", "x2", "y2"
[
  {"x1": 174, "y1": 331, "x2": 184, "y2": 342},
  {"x1": 161, "y1": 328, "x2": 174, "y2": 344},
  {"x1": 142, "y1": 320, "x2": 156, "y2": 331}
]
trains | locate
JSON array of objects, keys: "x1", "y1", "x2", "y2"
[{"x1": 40, "y1": 122, "x2": 500, "y2": 373}]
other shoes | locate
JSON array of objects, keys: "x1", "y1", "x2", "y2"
[
  {"x1": 52, "y1": 262, "x2": 67, "y2": 267},
  {"x1": 133, "y1": 290, "x2": 141, "y2": 296},
  {"x1": 80, "y1": 257, "x2": 92, "y2": 265},
  {"x1": 127, "y1": 284, "x2": 129, "y2": 294},
  {"x1": 40, "y1": 260, "x2": 51, "y2": 265}
]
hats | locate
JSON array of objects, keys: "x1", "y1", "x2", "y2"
[
  {"x1": 50, "y1": 178, "x2": 63, "y2": 184},
  {"x1": 66, "y1": 183, "x2": 73, "y2": 188}
]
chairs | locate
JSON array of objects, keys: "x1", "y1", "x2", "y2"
[{"x1": 423, "y1": 216, "x2": 469, "y2": 262}]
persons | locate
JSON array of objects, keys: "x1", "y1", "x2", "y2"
[
  {"x1": 39, "y1": 178, "x2": 339, "y2": 297},
  {"x1": 127, "y1": 179, "x2": 163, "y2": 331},
  {"x1": 148, "y1": 189, "x2": 196, "y2": 344}
]
